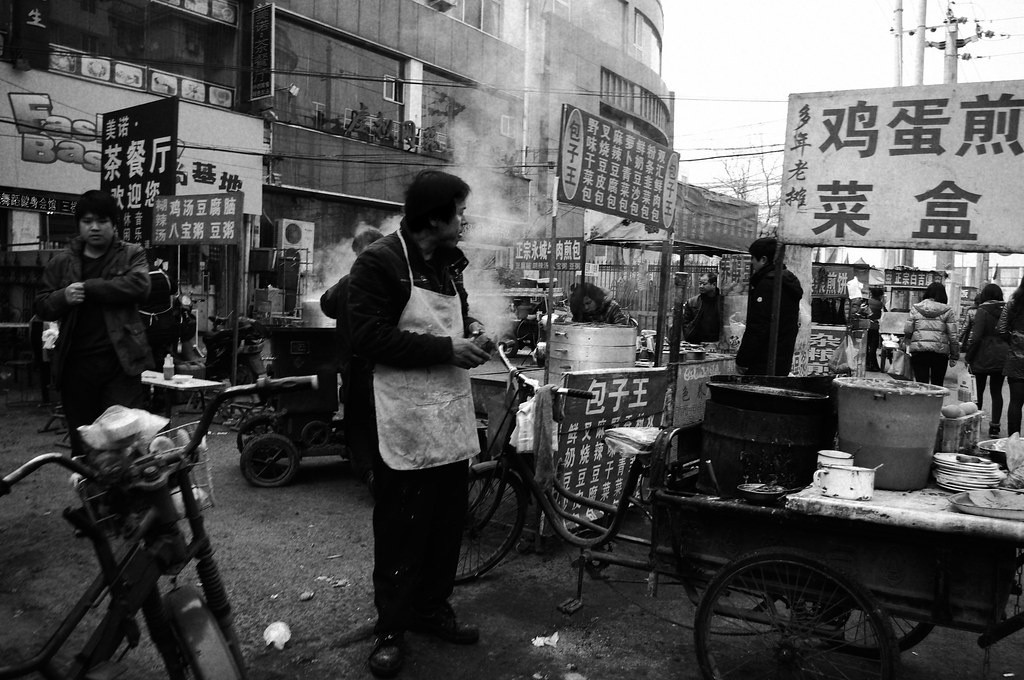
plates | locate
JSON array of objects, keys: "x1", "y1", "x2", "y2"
[{"x1": 930, "y1": 450, "x2": 1024, "y2": 519}]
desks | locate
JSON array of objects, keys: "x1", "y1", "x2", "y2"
[{"x1": 141, "y1": 370, "x2": 226, "y2": 429}]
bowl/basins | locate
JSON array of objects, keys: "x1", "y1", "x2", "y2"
[
  {"x1": 172, "y1": 374, "x2": 193, "y2": 384},
  {"x1": 737, "y1": 484, "x2": 786, "y2": 503},
  {"x1": 683, "y1": 351, "x2": 707, "y2": 361},
  {"x1": 977, "y1": 438, "x2": 1024, "y2": 462},
  {"x1": 663, "y1": 352, "x2": 687, "y2": 364}
]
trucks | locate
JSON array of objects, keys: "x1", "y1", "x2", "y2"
[{"x1": 466, "y1": 278, "x2": 564, "y2": 357}]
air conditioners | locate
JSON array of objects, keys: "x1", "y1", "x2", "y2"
[{"x1": 274, "y1": 218, "x2": 314, "y2": 272}]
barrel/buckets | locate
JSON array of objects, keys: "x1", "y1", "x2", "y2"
[
  {"x1": 832, "y1": 375, "x2": 950, "y2": 490},
  {"x1": 698, "y1": 399, "x2": 837, "y2": 499}
]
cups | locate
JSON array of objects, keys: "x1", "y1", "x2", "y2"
[
  {"x1": 813, "y1": 465, "x2": 874, "y2": 501},
  {"x1": 816, "y1": 450, "x2": 854, "y2": 474}
]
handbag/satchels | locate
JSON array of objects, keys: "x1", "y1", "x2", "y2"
[
  {"x1": 958, "y1": 364, "x2": 977, "y2": 403},
  {"x1": 828, "y1": 334, "x2": 859, "y2": 374},
  {"x1": 887, "y1": 335, "x2": 913, "y2": 382}
]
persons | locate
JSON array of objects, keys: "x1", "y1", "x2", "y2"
[
  {"x1": 904, "y1": 282, "x2": 960, "y2": 386},
  {"x1": 958, "y1": 284, "x2": 1009, "y2": 439},
  {"x1": 866, "y1": 288, "x2": 888, "y2": 372},
  {"x1": 735, "y1": 237, "x2": 803, "y2": 376},
  {"x1": 34, "y1": 190, "x2": 178, "y2": 527},
  {"x1": 682, "y1": 272, "x2": 724, "y2": 345},
  {"x1": 570, "y1": 283, "x2": 626, "y2": 325},
  {"x1": 320, "y1": 169, "x2": 498, "y2": 680},
  {"x1": 994, "y1": 275, "x2": 1024, "y2": 436}
]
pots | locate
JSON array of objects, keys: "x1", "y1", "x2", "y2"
[{"x1": 705, "y1": 380, "x2": 831, "y2": 414}]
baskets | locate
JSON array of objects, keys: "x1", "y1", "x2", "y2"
[{"x1": 70, "y1": 421, "x2": 216, "y2": 537}]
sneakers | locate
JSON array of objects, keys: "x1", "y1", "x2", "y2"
[
  {"x1": 367, "y1": 623, "x2": 408, "y2": 676},
  {"x1": 406, "y1": 604, "x2": 480, "y2": 645}
]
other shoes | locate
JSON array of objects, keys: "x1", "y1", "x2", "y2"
[{"x1": 74, "y1": 528, "x2": 88, "y2": 537}]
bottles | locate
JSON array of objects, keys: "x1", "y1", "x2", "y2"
[{"x1": 163, "y1": 354, "x2": 175, "y2": 380}]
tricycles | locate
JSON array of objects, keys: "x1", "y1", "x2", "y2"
[
  {"x1": 455, "y1": 342, "x2": 1024, "y2": 680},
  {"x1": 0, "y1": 368, "x2": 317, "y2": 680},
  {"x1": 619, "y1": 304, "x2": 638, "y2": 326},
  {"x1": 235, "y1": 361, "x2": 362, "y2": 488}
]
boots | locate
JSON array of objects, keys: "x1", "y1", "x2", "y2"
[{"x1": 989, "y1": 421, "x2": 1000, "y2": 437}]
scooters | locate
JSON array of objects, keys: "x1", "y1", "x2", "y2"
[{"x1": 201, "y1": 312, "x2": 267, "y2": 382}]
